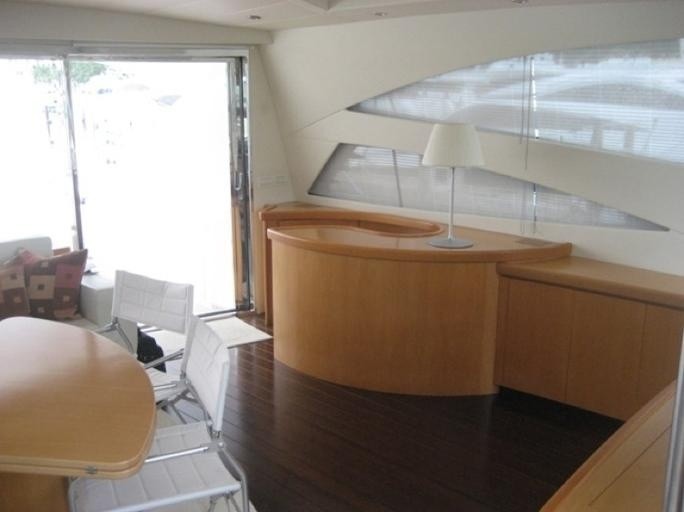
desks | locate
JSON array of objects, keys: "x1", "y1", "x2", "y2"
[
  {"x1": 258, "y1": 201, "x2": 572, "y2": 397},
  {"x1": 537, "y1": 379, "x2": 678, "y2": 511},
  {"x1": 494, "y1": 256, "x2": 684, "y2": 423}
]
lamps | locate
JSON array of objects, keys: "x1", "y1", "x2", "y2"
[{"x1": 421, "y1": 122, "x2": 485, "y2": 250}]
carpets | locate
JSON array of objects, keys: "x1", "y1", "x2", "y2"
[{"x1": 143, "y1": 316, "x2": 274, "y2": 361}]
